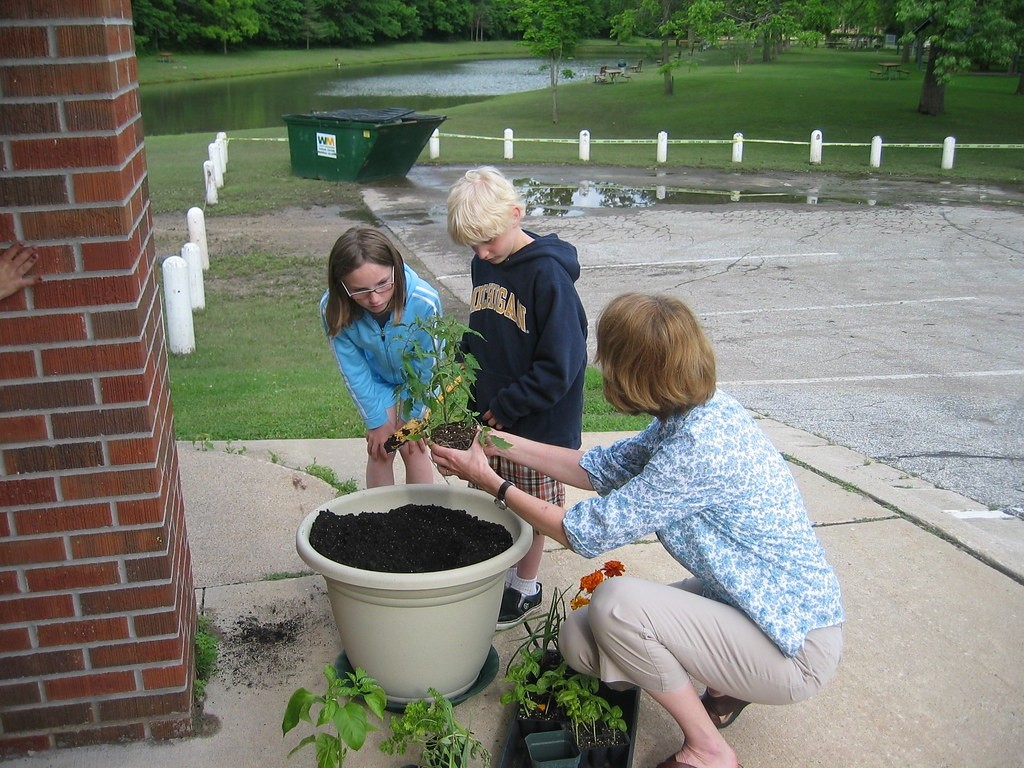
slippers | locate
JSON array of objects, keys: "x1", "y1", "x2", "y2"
[
  {"x1": 656, "y1": 753, "x2": 745, "y2": 768},
  {"x1": 701, "y1": 686, "x2": 751, "y2": 728}
]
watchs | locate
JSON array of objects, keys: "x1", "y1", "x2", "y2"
[{"x1": 494, "y1": 481, "x2": 515, "y2": 511}]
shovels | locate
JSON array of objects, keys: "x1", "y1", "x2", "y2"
[{"x1": 384, "y1": 373, "x2": 464, "y2": 454}]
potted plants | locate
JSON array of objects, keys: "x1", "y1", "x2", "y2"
[
  {"x1": 378, "y1": 688, "x2": 492, "y2": 768},
  {"x1": 500, "y1": 648, "x2": 630, "y2": 768},
  {"x1": 388, "y1": 315, "x2": 513, "y2": 450}
]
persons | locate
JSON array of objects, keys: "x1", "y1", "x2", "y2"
[
  {"x1": 0, "y1": 242, "x2": 44, "y2": 300},
  {"x1": 447, "y1": 165, "x2": 588, "y2": 630},
  {"x1": 320, "y1": 228, "x2": 444, "y2": 490},
  {"x1": 427, "y1": 291, "x2": 844, "y2": 768}
]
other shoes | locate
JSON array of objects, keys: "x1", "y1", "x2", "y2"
[{"x1": 496, "y1": 567, "x2": 542, "y2": 630}]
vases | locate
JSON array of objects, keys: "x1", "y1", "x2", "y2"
[
  {"x1": 296, "y1": 484, "x2": 534, "y2": 713},
  {"x1": 524, "y1": 731, "x2": 581, "y2": 768}
]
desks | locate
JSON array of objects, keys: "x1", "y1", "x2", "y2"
[{"x1": 605, "y1": 70, "x2": 622, "y2": 82}]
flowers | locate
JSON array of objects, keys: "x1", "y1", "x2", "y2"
[{"x1": 505, "y1": 561, "x2": 625, "y2": 676}]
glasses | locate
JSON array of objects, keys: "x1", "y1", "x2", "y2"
[{"x1": 341, "y1": 266, "x2": 394, "y2": 299}]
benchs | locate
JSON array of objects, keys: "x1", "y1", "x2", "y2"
[
  {"x1": 598, "y1": 75, "x2": 607, "y2": 84},
  {"x1": 657, "y1": 56, "x2": 664, "y2": 67},
  {"x1": 674, "y1": 51, "x2": 681, "y2": 59},
  {"x1": 623, "y1": 75, "x2": 631, "y2": 82},
  {"x1": 594, "y1": 66, "x2": 607, "y2": 82},
  {"x1": 630, "y1": 60, "x2": 642, "y2": 72},
  {"x1": 688, "y1": 48, "x2": 693, "y2": 56}
]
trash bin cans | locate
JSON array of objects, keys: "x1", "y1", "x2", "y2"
[
  {"x1": 617, "y1": 63, "x2": 627, "y2": 74},
  {"x1": 280, "y1": 108, "x2": 449, "y2": 184}
]
